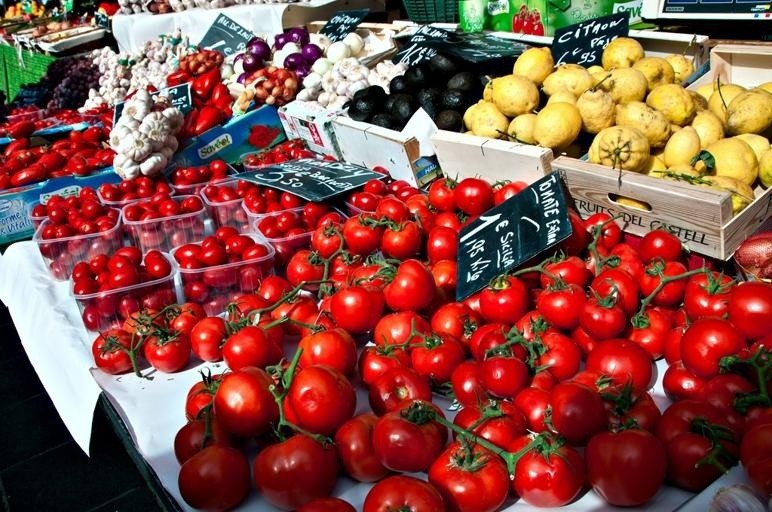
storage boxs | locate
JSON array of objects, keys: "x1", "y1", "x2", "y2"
[
  {"x1": 277, "y1": 23, "x2": 458, "y2": 163},
  {"x1": 434, "y1": 30, "x2": 707, "y2": 200},
  {"x1": 23, "y1": 202, "x2": 57, "y2": 230},
  {"x1": 69, "y1": 268, "x2": 178, "y2": 332},
  {"x1": 32, "y1": 209, "x2": 122, "y2": 279},
  {"x1": 552, "y1": 43, "x2": 772, "y2": 261},
  {"x1": 120, "y1": 195, "x2": 206, "y2": 259},
  {"x1": 201, "y1": 186, "x2": 245, "y2": 230},
  {"x1": 332, "y1": 30, "x2": 547, "y2": 202},
  {"x1": 172, "y1": 243, "x2": 273, "y2": 318},
  {"x1": 251, "y1": 216, "x2": 309, "y2": 259},
  {"x1": 159, "y1": 105, "x2": 285, "y2": 184},
  {"x1": 0, "y1": 170, "x2": 130, "y2": 244}
]
